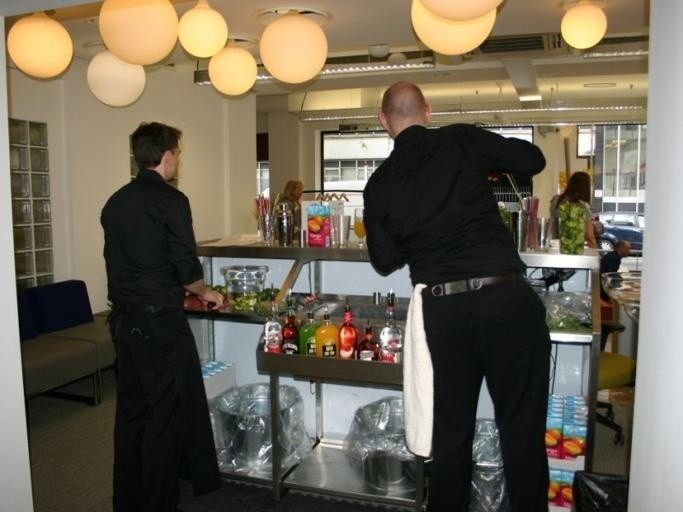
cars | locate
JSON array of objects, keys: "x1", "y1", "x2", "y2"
[{"x1": 595, "y1": 209, "x2": 643, "y2": 254}]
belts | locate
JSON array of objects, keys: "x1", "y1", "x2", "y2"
[
  {"x1": 423, "y1": 275, "x2": 516, "y2": 298},
  {"x1": 110, "y1": 303, "x2": 161, "y2": 315}
]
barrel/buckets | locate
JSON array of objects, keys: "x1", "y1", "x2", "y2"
[{"x1": 355, "y1": 397, "x2": 420, "y2": 493}]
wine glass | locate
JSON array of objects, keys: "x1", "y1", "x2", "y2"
[{"x1": 352, "y1": 207, "x2": 367, "y2": 250}]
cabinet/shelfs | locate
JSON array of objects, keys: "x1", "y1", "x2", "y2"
[{"x1": 173, "y1": 294, "x2": 591, "y2": 512}]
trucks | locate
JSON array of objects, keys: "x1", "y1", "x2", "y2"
[{"x1": 595, "y1": 197, "x2": 644, "y2": 225}]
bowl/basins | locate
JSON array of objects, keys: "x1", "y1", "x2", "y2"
[
  {"x1": 598, "y1": 269, "x2": 641, "y2": 305},
  {"x1": 355, "y1": 397, "x2": 430, "y2": 498}
]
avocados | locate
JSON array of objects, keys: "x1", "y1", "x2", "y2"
[
  {"x1": 206, "y1": 284, "x2": 227, "y2": 296},
  {"x1": 261, "y1": 288, "x2": 280, "y2": 300}
]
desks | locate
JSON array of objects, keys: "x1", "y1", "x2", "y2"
[{"x1": 598, "y1": 269, "x2": 641, "y2": 373}]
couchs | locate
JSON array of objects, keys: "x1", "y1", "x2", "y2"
[{"x1": 18, "y1": 276, "x2": 113, "y2": 406}]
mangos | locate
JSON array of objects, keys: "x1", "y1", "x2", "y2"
[
  {"x1": 563, "y1": 441, "x2": 583, "y2": 456},
  {"x1": 544, "y1": 432, "x2": 558, "y2": 447},
  {"x1": 548, "y1": 489, "x2": 556, "y2": 500},
  {"x1": 309, "y1": 220, "x2": 320, "y2": 232},
  {"x1": 562, "y1": 488, "x2": 573, "y2": 501}
]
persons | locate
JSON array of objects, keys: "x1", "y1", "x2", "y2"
[
  {"x1": 600, "y1": 239, "x2": 631, "y2": 304},
  {"x1": 361, "y1": 81, "x2": 550, "y2": 512},
  {"x1": 549, "y1": 171, "x2": 597, "y2": 248},
  {"x1": 98, "y1": 122, "x2": 225, "y2": 512}
]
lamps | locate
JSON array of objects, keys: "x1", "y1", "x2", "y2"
[
  {"x1": 83, "y1": 49, "x2": 150, "y2": 106},
  {"x1": 206, "y1": 44, "x2": 260, "y2": 99},
  {"x1": 558, "y1": 4, "x2": 608, "y2": 52},
  {"x1": 257, "y1": 13, "x2": 331, "y2": 86},
  {"x1": 173, "y1": 2, "x2": 232, "y2": 60},
  {"x1": 408, "y1": 2, "x2": 500, "y2": 58},
  {"x1": 421, "y1": 1, "x2": 502, "y2": 24},
  {"x1": 95, "y1": 1, "x2": 181, "y2": 68},
  {"x1": 5, "y1": 2, "x2": 77, "y2": 83}
]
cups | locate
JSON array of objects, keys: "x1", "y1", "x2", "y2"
[
  {"x1": 520, "y1": 195, "x2": 540, "y2": 250},
  {"x1": 534, "y1": 217, "x2": 555, "y2": 252},
  {"x1": 257, "y1": 214, "x2": 276, "y2": 247},
  {"x1": 221, "y1": 263, "x2": 269, "y2": 312},
  {"x1": 506, "y1": 208, "x2": 531, "y2": 254},
  {"x1": 330, "y1": 214, "x2": 351, "y2": 247}
]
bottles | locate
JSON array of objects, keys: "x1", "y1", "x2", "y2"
[
  {"x1": 275, "y1": 201, "x2": 293, "y2": 246},
  {"x1": 263, "y1": 282, "x2": 401, "y2": 365}
]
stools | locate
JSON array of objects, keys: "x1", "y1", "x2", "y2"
[{"x1": 590, "y1": 320, "x2": 633, "y2": 445}]
